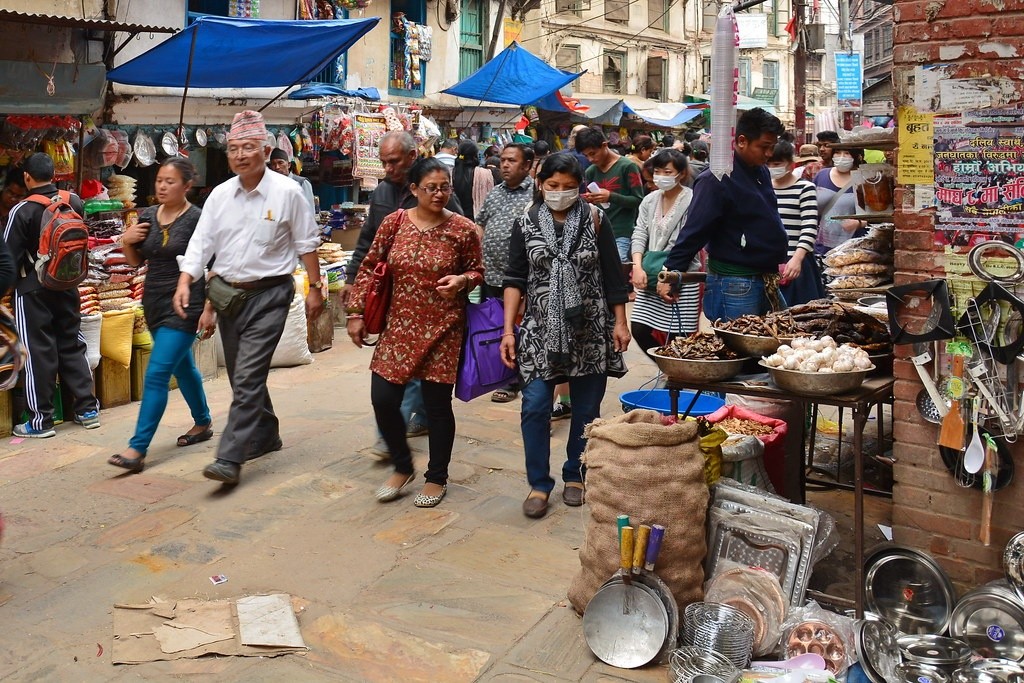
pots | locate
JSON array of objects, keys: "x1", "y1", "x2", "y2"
[
  {"x1": 582, "y1": 514, "x2": 679, "y2": 672},
  {"x1": 940, "y1": 385, "x2": 1014, "y2": 493}
]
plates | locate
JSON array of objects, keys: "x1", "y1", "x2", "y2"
[{"x1": 708, "y1": 565, "x2": 782, "y2": 655}]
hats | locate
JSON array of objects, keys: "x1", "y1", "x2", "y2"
[
  {"x1": 229, "y1": 109, "x2": 267, "y2": 140},
  {"x1": 270, "y1": 148, "x2": 288, "y2": 160},
  {"x1": 792, "y1": 144, "x2": 818, "y2": 163}
]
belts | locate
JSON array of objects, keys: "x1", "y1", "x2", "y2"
[{"x1": 223, "y1": 273, "x2": 291, "y2": 289}]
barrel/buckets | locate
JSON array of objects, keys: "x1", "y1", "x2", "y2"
[{"x1": 618, "y1": 388, "x2": 725, "y2": 417}]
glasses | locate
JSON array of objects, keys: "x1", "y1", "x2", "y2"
[
  {"x1": 416, "y1": 185, "x2": 454, "y2": 195},
  {"x1": 226, "y1": 144, "x2": 268, "y2": 158}
]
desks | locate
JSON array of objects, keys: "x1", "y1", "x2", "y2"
[{"x1": 662, "y1": 364, "x2": 894, "y2": 622}]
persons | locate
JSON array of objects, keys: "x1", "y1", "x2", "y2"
[
  {"x1": 884, "y1": 150, "x2": 893, "y2": 164},
  {"x1": 170, "y1": 111, "x2": 323, "y2": 479},
  {"x1": 791, "y1": 143, "x2": 822, "y2": 178},
  {"x1": 660, "y1": 135, "x2": 674, "y2": 149},
  {"x1": 625, "y1": 135, "x2": 655, "y2": 173},
  {"x1": 766, "y1": 132, "x2": 825, "y2": 308},
  {"x1": 342, "y1": 157, "x2": 485, "y2": 509},
  {"x1": 525, "y1": 156, "x2": 571, "y2": 436},
  {"x1": 475, "y1": 144, "x2": 540, "y2": 405},
  {"x1": 681, "y1": 139, "x2": 709, "y2": 188},
  {"x1": 269, "y1": 147, "x2": 316, "y2": 270},
  {"x1": 109, "y1": 157, "x2": 218, "y2": 472},
  {"x1": 631, "y1": 141, "x2": 708, "y2": 364},
  {"x1": 1, "y1": 233, "x2": 16, "y2": 300},
  {"x1": 812, "y1": 139, "x2": 869, "y2": 257},
  {"x1": 802, "y1": 131, "x2": 840, "y2": 181},
  {"x1": 656, "y1": 108, "x2": 789, "y2": 335},
  {"x1": 499, "y1": 150, "x2": 631, "y2": 518},
  {"x1": 575, "y1": 126, "x2": 646, "y2": 277},
  {"x1": 337, "y1": 129, "x2": 464, "y2": 458},
  {"x1": 642, "y1": 156, "x2": 659, "y2": 191},
  {"x1": 5, "y1": 153, "x2": 101, "y2": 437}
]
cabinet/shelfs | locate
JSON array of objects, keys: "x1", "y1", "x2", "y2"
[{"x1": 824, "y1": 138, "x2": 896, "y2": 294}]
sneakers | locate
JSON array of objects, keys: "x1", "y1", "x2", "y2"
[
  {"x1": 74, "y1": 410, "x2": 100, "y2": 429},
  {"x1": 550, "y1": 401, "x2": 572, "y2": 420},
  {"x1": 12, "y1": 420, "x2": 56, "y2": 438}
]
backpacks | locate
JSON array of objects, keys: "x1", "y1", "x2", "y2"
[{"x1": 23, "y1": 190, "x2": 91, "y2": 291}]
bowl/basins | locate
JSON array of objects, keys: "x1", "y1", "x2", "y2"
[
  {"x1": 710, "y1": 327, "x2": 797, "y2": 362},
  {"x1": 759, "y1": 358, "x2": 876, "y2": 394},
  {"x1": 645, "y1": 346, "x2": 751, "y2": 384},
  {"x1": 857, "y1": 296, "x2": 888, "y2": 310},
  {"x1": 852, "y1": 530, "x2": 1024, "y2": 681}
]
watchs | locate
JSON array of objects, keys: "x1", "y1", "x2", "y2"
[{"x1": 309, "y1": 280, "x2": 323, "y2": 289}]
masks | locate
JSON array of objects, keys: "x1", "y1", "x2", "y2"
[
  {"x1": 653, "y1": 171, "x2": 681, "y2": 191},
  {"x1": 767, "y1": 161, "x2": 792, "y2": 179},
  {"x1": 543, "y1": 183, "x2": 580, "y2": 211},
  {"x1": 832, "y1": 157, "x2": 854, "y2": 172}
]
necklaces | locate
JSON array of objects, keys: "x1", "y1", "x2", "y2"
[{"x1": 159, "y1": 201, "x2": 186, "y2": 248}]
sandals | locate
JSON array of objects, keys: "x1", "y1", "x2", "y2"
[
  {"x1": 177, "y1": 421, "x2": 213, "y2": 447},
  {"x1": 108, "y1": 452, "x2": 146, "y2": 471},
  {"x1": 491, "y1": 389, "x2": 517, "y2": 402}
]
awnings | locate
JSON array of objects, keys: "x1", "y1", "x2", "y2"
[
  {"x1": 567, "y1": 96, "x2": 709, "y2": 129},
  {"x1": 0, "y1": 7, "x2": 182, "y2": 61},
  {"x1": 439, "y1": 39, "x2": 589, "y2": 131},
  {"x1": 108, "y1": 13, "x2": 382, "y2": 147}
]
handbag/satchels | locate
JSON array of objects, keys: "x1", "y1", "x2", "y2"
[
  {"x1": 363, "y1": 258, "x2": 392, "y2": 334},
  {"x1": 208, "y1": 274, "x2": 247, "y2": 319},
  {"x1": 455, "y1": 277, "x2": 520, "y2": 402},
  {"x1": 641, "y1": 251, "x2": 682, "y2": 296}
]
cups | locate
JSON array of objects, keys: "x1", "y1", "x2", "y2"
[
  {"x1": 859, "y1": 162, "x2": 894, "y2": 214},
  {"x1": 851, "y1": 169, "x2": 866, "y2": 216}
]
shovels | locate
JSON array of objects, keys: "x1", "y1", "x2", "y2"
[
  {"x1": 980, "y1": 446, "x2": 991, "y2": 547},
  {"x1": 938, "y1": 354, "x2": 965, "y2": 450}
]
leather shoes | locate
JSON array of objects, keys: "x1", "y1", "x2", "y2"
[
  {"x1": 202, "y1": 459, "x2": 240, "y2": 484},
  {"x1": 245, "y1": 433, "x2": 282, "y2": 459}
]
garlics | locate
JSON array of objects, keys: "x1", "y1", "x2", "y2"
[{"x1": 761, "y1": 335, "x2": 872, "y2": 373}]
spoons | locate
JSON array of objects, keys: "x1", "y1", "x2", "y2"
[{"x1": 747, "y1": 652, "x2": 827, "y2": 673}]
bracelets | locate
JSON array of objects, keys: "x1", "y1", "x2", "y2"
[
  {"x1": 501, "y1": 332, "x2": 514, "y2": 336},
  {"x1": 857, "y1": 218, "x2": 862, "y2": 228},
  {"x1": 457, "y1": 274, "x2": 467, "y2": 291},
  {"x1": 205, "y1": 298, "x2": 211, "y2": 303},
  {"x1": 344, "y1": 315, "x2": 364, "y2": 319}
]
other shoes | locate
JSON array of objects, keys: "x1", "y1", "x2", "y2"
[
  {"x1": 372, "y1": 436, "x2": 392, "y2": 459},
  {"x1": 523, "y1": 486, "x2": 550, "y2": 517},
  {"x1": 413, "y1": 482, "x2": 447, "y2": 506},
  {"x1": 404, "y1": 414, "x2": 429, "y2": 438},
  {"x1": 375, "y1": 468, "x2": 415, "y2": 502},
  {"x1": 563, "y1": 482, "x2": 586, "y2": 506}
]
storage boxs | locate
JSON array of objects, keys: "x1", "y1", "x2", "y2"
[{"x1": 0, "y1": 328, "x2": 218, "y2": 439}]
